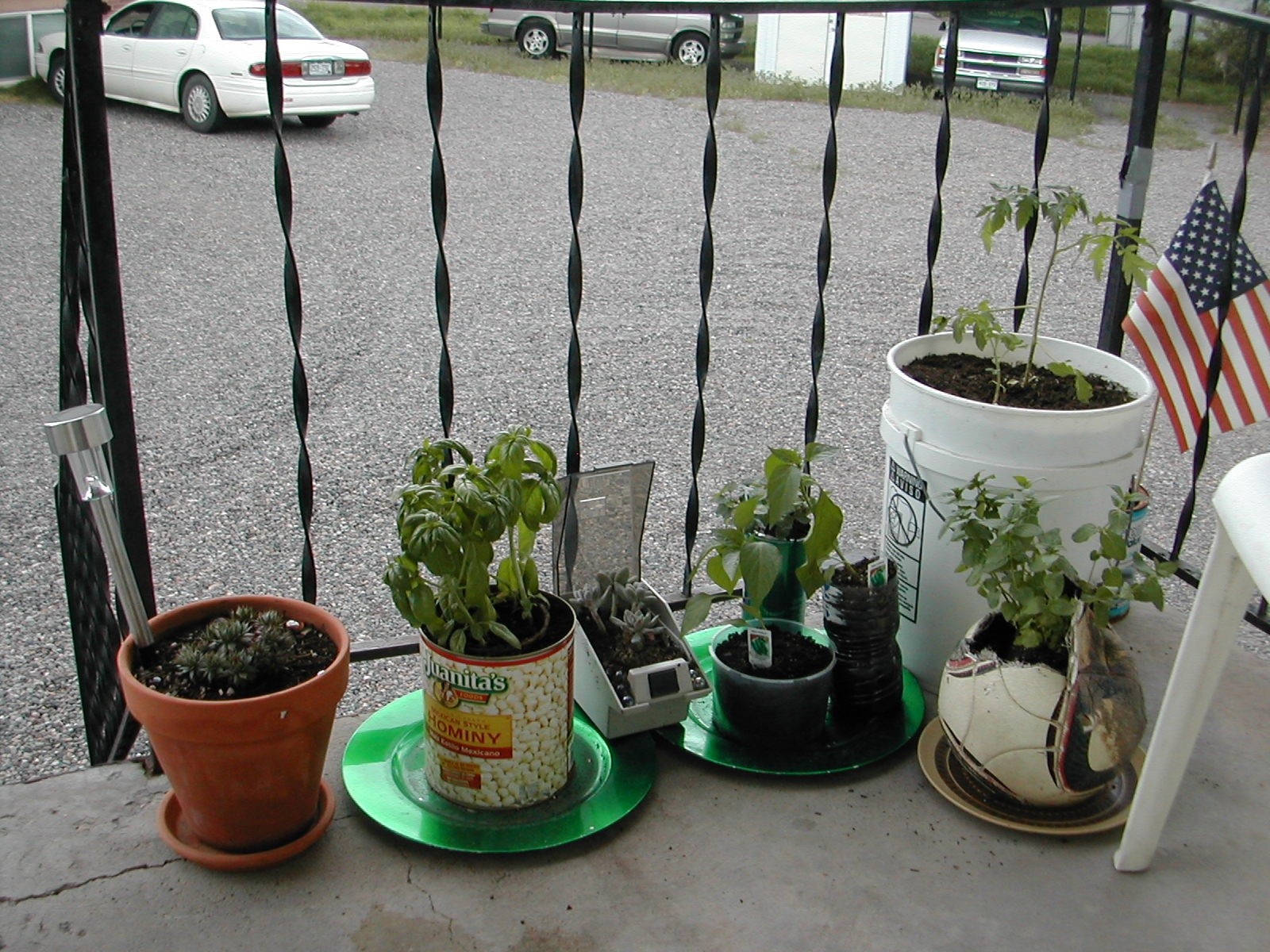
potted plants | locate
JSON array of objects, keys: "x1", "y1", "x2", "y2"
[
  {"x1": 115, "y1": 592, "x2": 351, "y2": 872},
  {"x1": 697, "y1": 181, "x2": 1192, "y2": 819},
  {"x1": 381, "y1": 428, "x2": 576, "y2": 805}
]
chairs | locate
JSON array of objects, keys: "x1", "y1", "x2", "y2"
[{"x1": 1113, "y1": 448, "x2": 1270, "y2": 872}]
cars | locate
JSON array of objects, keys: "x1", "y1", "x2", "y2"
[
  {"x1": 931, "y1": 7, "x2": 1052, "y2": 96},
  {"x1": 479, "y1": 9, "x2": 747, "y2": 68},
  {"x1": 32, "y1": 1, "x2": 376, "y2": 135}
]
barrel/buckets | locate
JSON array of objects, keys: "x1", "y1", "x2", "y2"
[{"x1": 881, "y1": 330, "x2": 1154, "y2": 693}]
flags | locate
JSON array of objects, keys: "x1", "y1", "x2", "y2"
[{"x1": 1120, "y1": 176, "x2": 1270, "y2": 454}]
